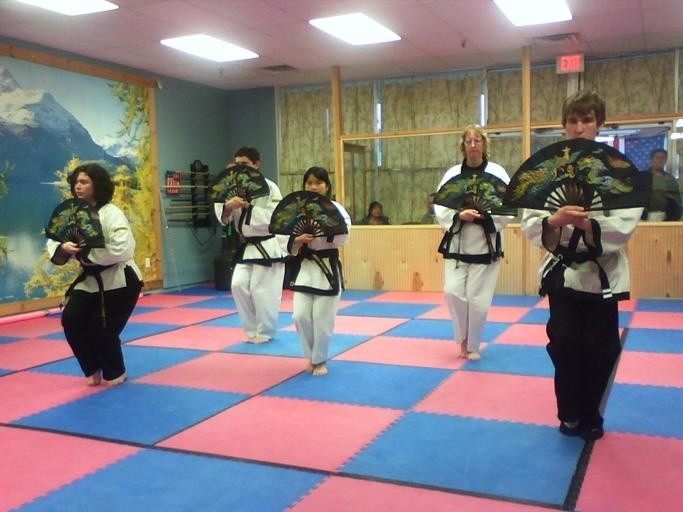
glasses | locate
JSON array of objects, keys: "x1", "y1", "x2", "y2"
[{"x1": 465, "y1": 139, "x2": 482, "y2": 145}]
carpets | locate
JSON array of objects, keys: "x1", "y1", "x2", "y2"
[{"x1": 0, "y1": 288, "x2": 683, "y2": 512}]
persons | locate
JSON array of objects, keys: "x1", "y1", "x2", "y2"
[
  {"x1": 421, "y1": 191, "x2": 436, "y2": 224},
  {"x1": 355, "y1": 200, "x2": 392, "y2": 226},
  {"x1": 45, "y1": 163, "x2": 146, "y2": 387},
  {"x1": 642, "y1": 148, "x2": 683, "y2": 221},
  {"x1": 275, "y1": 166, "x2": 352, "y2": 377},
  {"x1": 433, "y1": 125, "x2": 519, "y2": 362},
  {"x1": 213, "y1": 146, "x2": 289, "y2": 343},
  {"x1": 520, "y1": 91, "x2": 647, "y2": 442}
]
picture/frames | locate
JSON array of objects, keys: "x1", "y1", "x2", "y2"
[{"x1": 0, "y1": 43, "x2": 163, "y2": 318}]
